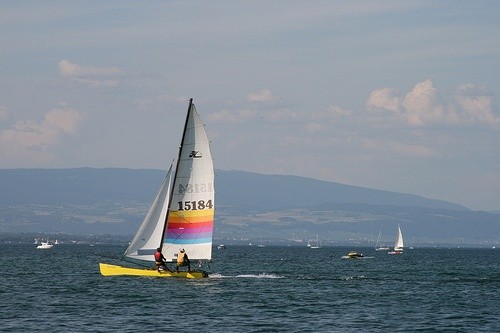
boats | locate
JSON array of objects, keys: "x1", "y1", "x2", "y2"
[
  {"x1": 347, "y1": 250, "x2": 365, "y2": 259},
  {"x1": 218, "y1": 245, "x2": 226, "y2": 249},
  {"x1": 35, "y1": 242, "x2": 54, "y2": 249}
]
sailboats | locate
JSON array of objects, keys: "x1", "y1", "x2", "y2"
[
  {"x1": 307, "y1": 234, "x2": 323, "y2": 249},
  {"x1": 375, "y1": 223, "x2": 404, "y2": 255},
  {"x1": 93, "y1": 96, "x2": 216, "y2": 279}
]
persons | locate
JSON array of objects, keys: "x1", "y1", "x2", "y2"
[
  {"x1": 176, "y1": 248, "x2": 192, "y2": 273},
  {"x1": 154, "y1": 248, "x2": 174, "y2": 273}
]
